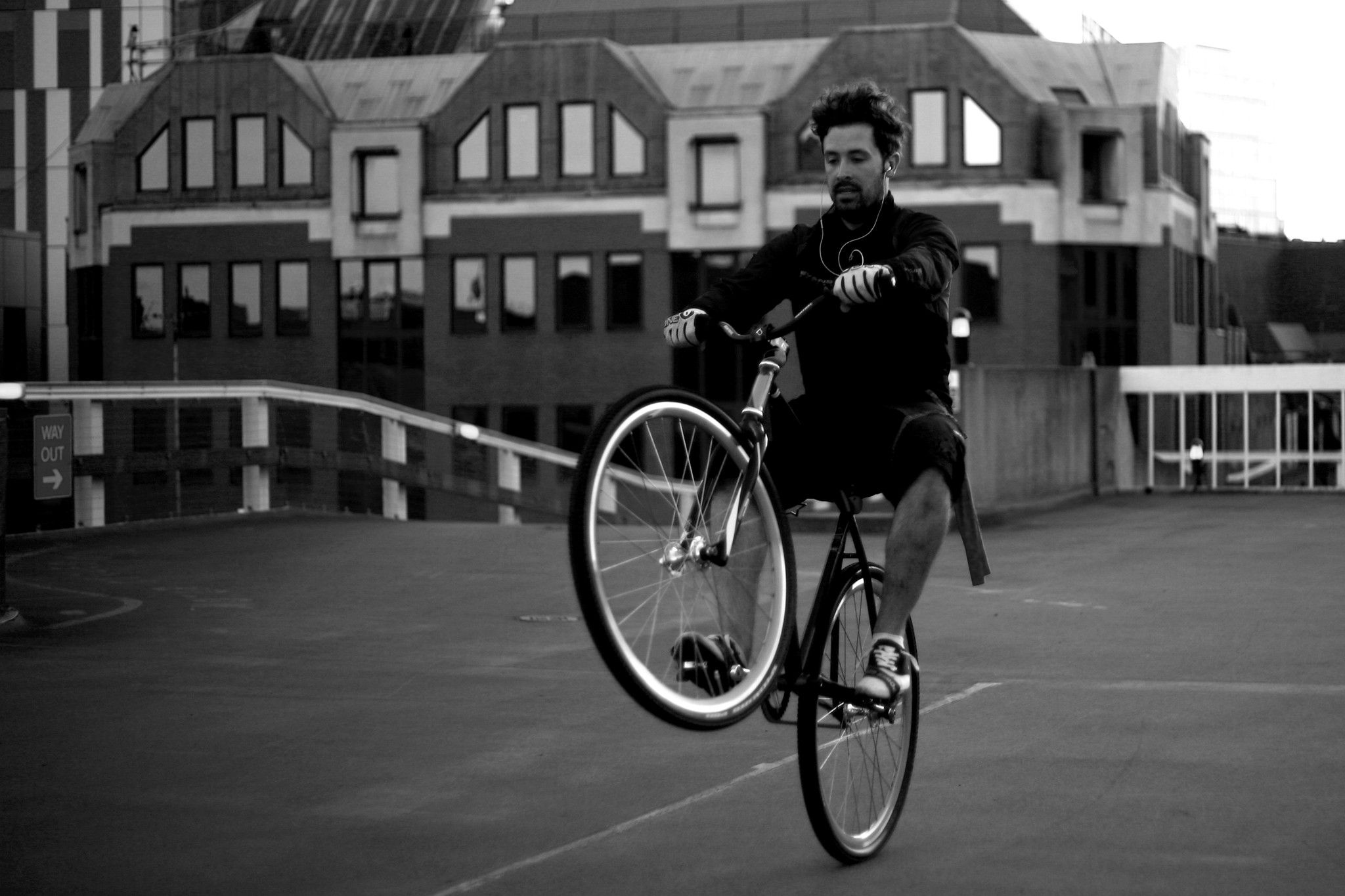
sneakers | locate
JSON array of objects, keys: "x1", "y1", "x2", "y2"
[
  {"x1": 673, "y1": 628, "x2": 751, "y2": 697},
  {"x1": 854, "y1": 637, "x2": 918, "y2": 699}
]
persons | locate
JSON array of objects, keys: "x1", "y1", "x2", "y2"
[{"x1": 663, "y1": 84, "x2": 991, "y2": 699}]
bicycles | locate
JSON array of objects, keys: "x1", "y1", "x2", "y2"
[{"x1": 561, "y1": 271, "x2": 928, "y2": 868}]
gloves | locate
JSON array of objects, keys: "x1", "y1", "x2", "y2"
[
  {"x1": 834, "y1": 265, "x2": 894, "y2": 312},
  {"x1": 662, "y1": 306, "x2": 710, "y2": 347}
]
